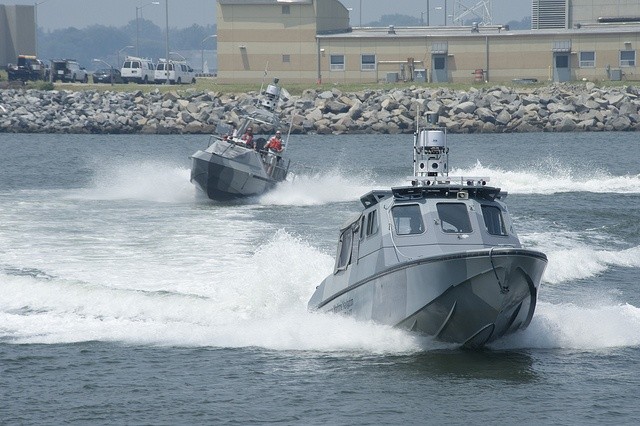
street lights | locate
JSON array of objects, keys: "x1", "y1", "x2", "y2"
[
  {"x1": 117, "y1": 45, "x2": 136, "y2": 72},
  {"x1": 201, "y1": 34, "x2": 220, "y2": 73},
  {"x1": 135, "y1": 0, "x2": 161, "y2": 56}
]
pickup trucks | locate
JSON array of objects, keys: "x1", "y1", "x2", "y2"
[{"x1": 7, "y1": 54, "x2": 49, "y2": 82}]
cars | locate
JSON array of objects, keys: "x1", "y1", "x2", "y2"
[{"x1": 91, "y1": 67, "x2": 128, "y2": 83}]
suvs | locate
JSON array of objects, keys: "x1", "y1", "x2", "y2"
[{"x1": 46, "y1": 57, "x2": 90, "y2": 83}]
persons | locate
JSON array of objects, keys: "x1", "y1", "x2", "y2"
[
  {"x1": 241, "y1": 127, "x2": 254, "y2": 149},
  {"x1": 409, "y1": 217, "x2": 422, "y2": 234},
  {"x1": 223, "y1": 134, "x2": 227, "y2": 141},
  {"x1": 264, "y1": 131, "x2": 285, "y2": 174}
]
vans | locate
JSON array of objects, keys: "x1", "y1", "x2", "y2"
[
  {"x1": 120, "y1": 55, "x2": 155, "y2": 84},
  {"x1": 153, "y1": 57, "x2": 196, "y2": 85}
]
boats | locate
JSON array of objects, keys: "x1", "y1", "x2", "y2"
[
  {"x1": 189, "y1": 61, "x2": 297, "y2": 203},
  {"x1": 307, "y1": 103, "x2": 548, "y2": 352}
]
